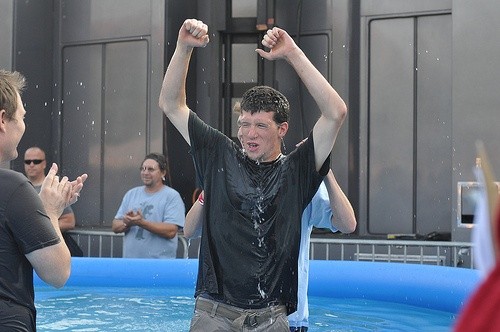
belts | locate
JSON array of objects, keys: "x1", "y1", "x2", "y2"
[
  {"x1": 195, "y1": 298, "x2": 287, "y2": 332},
  {"x1": 290, "y1": 326, "x2": 308, "y2": 332}
]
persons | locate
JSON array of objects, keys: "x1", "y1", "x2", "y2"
[
  {"x1": 182, "y1": 102, "x2": 357, "y2": 331},
  {"x1": 24, "y1": 146, "x2": 76, "y2": 231},
  {"x1": 112, "y1": 153, "x2": 185, "y2": 259},
  {"x1": 159, "y1": 18, "x2": 347, "y2": 331},
  {"x1": 0, "y1": 69, "x2": 88, "y2": 332}
]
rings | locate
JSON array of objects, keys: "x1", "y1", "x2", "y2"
[{"x1": 74, "y1": 192, "x2": 80, "y2": 198}]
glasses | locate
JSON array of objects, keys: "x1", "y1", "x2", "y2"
[
  {"x1": 24, "y1": 159, "x2": 45, "y2": 165},
  {"x1": 140, "y1": 166, "x2": 161, "y2": 172}
]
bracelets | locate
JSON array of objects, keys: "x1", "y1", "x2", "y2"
[{"x1": 198, "y1": 193, "x2": 204, "y2": 205}]
